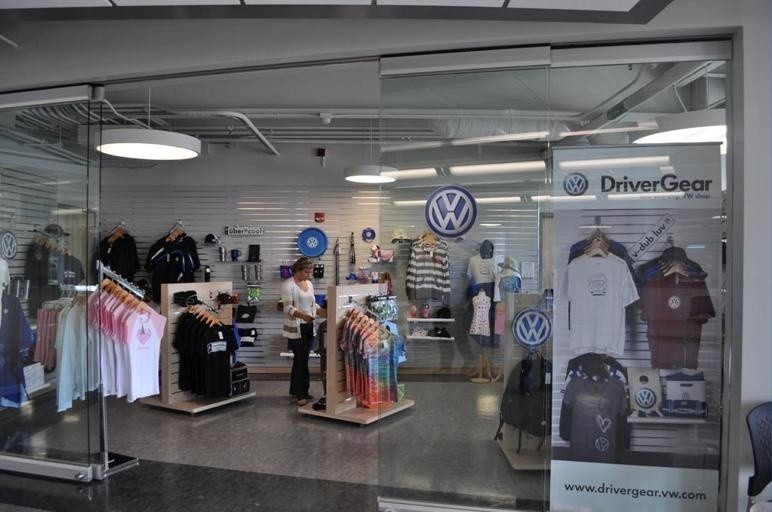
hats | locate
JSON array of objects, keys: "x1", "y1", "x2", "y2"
[
  {"x1": 391, "y1": 228, "x2": 411, "y2": 243},
  {"x1": 480, "y1": 240, "x2": 493, "y2": 258}
]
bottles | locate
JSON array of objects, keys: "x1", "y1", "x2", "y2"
[{"x1": 205, "y1": 266, "x2": 210, "y2": 281}]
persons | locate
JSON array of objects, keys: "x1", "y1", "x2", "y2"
[
  {"x1": 467, "y1": 239, "x2": 500, "y2": 350},
  {"x1": 280, "y1": 257, "x2": 328, "y2": 406}
]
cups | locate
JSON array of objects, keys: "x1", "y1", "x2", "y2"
[{"x1": 219, "y1": 247, "x2": 261, "y2": 281}]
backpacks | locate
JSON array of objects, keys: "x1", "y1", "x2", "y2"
[{"x1": 496, "y1": 350, "x2": 552, "y2": 453}]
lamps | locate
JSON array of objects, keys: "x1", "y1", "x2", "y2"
[
  {"x1": 629, "y1": 66, "x2": 727, "y2": 155},
  {"x1": 94, "y1": 85, "x2": 202, "y2": 161},
  {"x1": 343, "y1": 120, "x2": 399, "y2": 184}
]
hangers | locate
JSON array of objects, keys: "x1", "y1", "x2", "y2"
[
  {"x1": 165, "y1": 224, "x2": 184, "y2": 243},
  {"x1": 107, "y1": 222, "x2": 126, "y2": 244},
  {"x1": 582, "y1": 223, "x2": 610, "y2": 259},
  {"x1": 70, "y1": 282, "x2": 88, "y2": 306},
  {"x1": 185, "y1": 295, "x2": 223, "y2": 329},
  {"x1": 348, "y1": 300, "x2": 392, "y2": 337},
  {"x1": 422, "y1": 226, "x2": 438, "y2": 246},
  {"x1": 656, "y1": 236, "x2": 691, "y2": 279},
  {"x1": 99, "y1": 265, "x2": 152, "y2": 316},
  {"x1": 35, "y1": 229, "x2": 72, "y2": 256}
]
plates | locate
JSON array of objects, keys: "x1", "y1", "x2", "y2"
[{"x1": 297, "y1": 227, "x2": 328, "y2": 257}]
